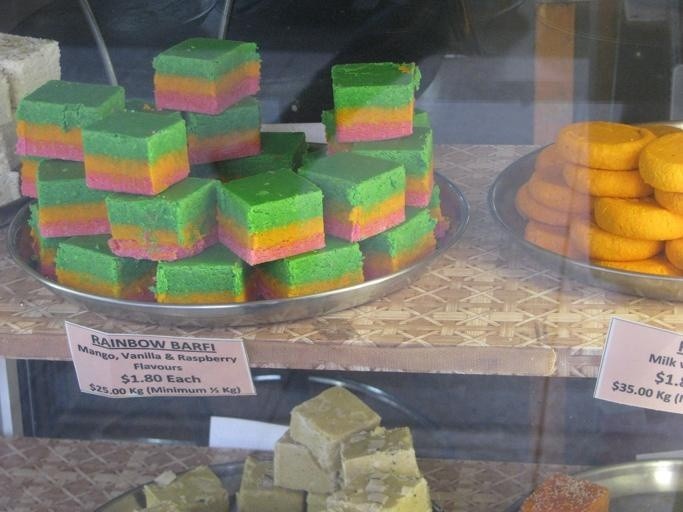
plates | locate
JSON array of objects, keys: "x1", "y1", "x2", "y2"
[
  {"x1": 0, "y1": 141, "x2": 472, "y2": 328},
  {"x1": 94, "y1": 460, "x2": 445, "y2": 512},
  {"x1": 503, "y1": 457, "x2": 683, "y2": 512},
  {"x1": 489, "y1": 142, "x2": 683, "y2": 302}
]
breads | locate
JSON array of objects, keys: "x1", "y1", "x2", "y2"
[
  {"x1": 518, "y1": 471, "x2": 611, "y2": 511},
  {"x1": 514, "y1": 117, "x2": 683, "y2": 276}
]
